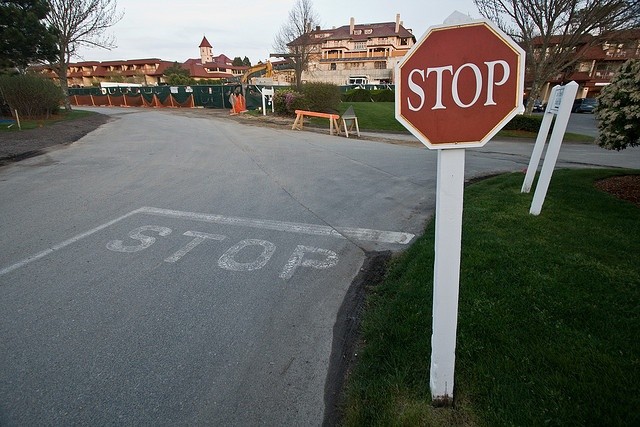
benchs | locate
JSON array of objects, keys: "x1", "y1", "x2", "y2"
[{"x1": 291, "y1": 110, "x2": 344, "y2": 136}]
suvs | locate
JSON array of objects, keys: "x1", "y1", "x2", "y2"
[{"x1": 573, "y1": 99, "x2": 595, "y2": 114}]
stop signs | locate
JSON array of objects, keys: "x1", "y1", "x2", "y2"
[{"x1": 394, "y1": 18, "x2": 526, "y2": 150}]
